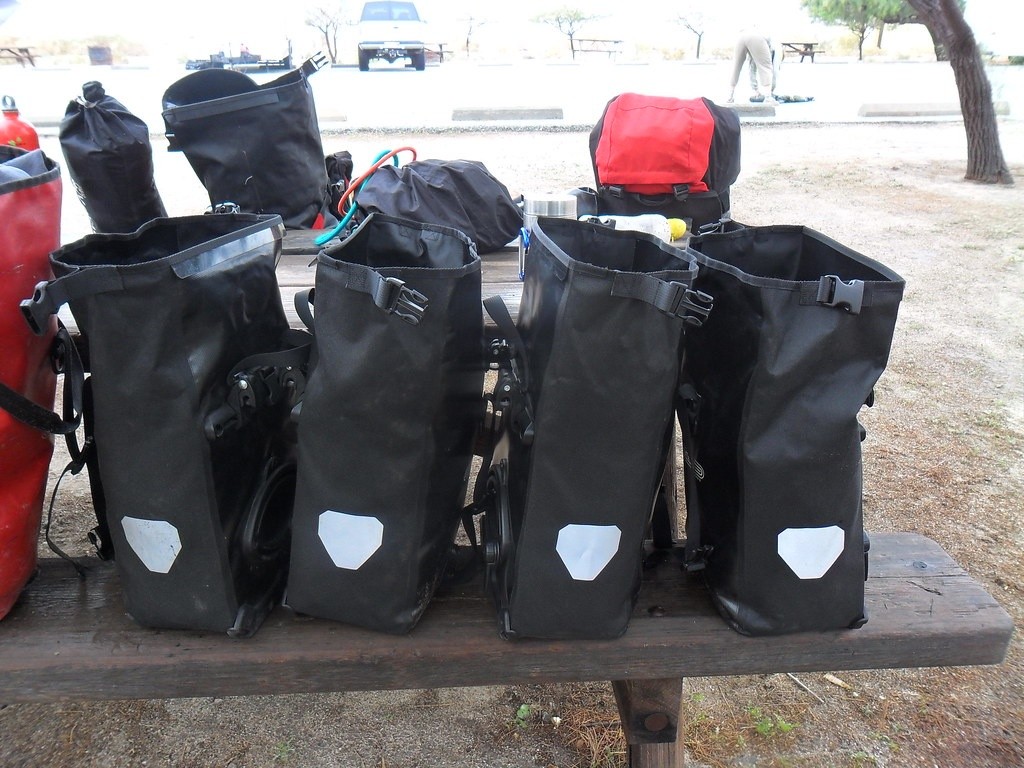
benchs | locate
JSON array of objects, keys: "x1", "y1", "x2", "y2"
[
  {"x1": 1, "y1": 526, "x2": 1014, "y2": 768},
  {"x1": 782, "y1": 51, "x2": 826, "y2": 55},
  {"x1": 266, "y1": 251, "x2": 527, "y2": 286},
  {"x1": 259, "y1": 61, "x2": 285, "y2": 72}
]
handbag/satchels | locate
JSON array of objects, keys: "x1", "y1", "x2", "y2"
[{"x1": 0, "y1": 50, "x2": 906, "y2": 639}]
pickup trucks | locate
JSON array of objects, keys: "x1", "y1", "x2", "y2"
[{"x1": 357, "y1": 1, "x2": 426, "y2": 72}]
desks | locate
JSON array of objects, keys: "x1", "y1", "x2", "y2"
[{"x1": 781, "y1": 42, "x2": 818, "y2": 63}]
